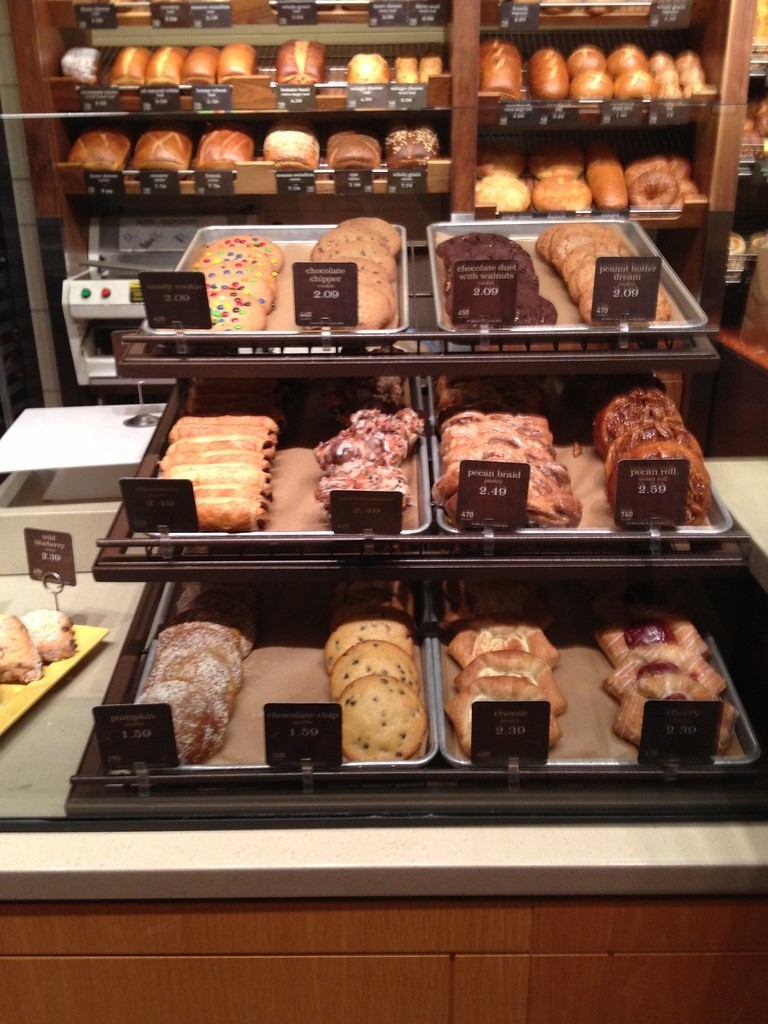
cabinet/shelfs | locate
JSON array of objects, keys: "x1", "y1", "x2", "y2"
[{"x1": 8, "y1": 0, "x2": 758, "y2": 458}]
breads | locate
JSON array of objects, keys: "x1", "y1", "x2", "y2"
[
  {"x1": 158, "y1": 372, "x2": 713, "y2": 530},
  {"x1": 135, "y1": 570, "x2": 262, "y2": 767},
  {"x1": 187, "y1": 216, "x2": 672, "y2": 329},
  {"x1": 0, "y1": 608, "x2": 77, "y2": 684},
  {"x1": 58, "y1": 40, "x2": 714, "y2": 215},
  {"x1": 323, "y1": 573, "x2": 739, "y2": 763}
]
